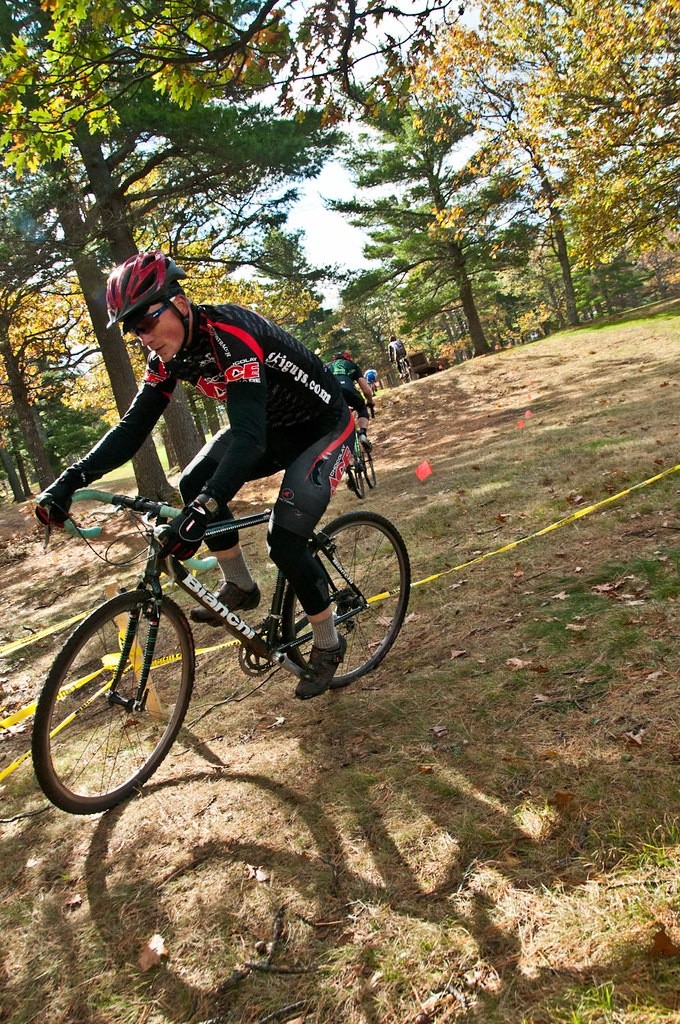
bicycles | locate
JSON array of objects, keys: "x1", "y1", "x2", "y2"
[
  {"x1": 343, "y1": 404, "x2": 378, "y2": 500},
  {"x1": 364, "y1": 377, "x2": 378, "y2": 397},
  {"x1": 30, "y1": 488, "x2": 411, "y2": 816},
  {"x1": 388, "y1": 354, "x2": 413, "y2": 384}
]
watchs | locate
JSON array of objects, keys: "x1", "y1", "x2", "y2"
[{"x1": 196, "y1": 494, "x2": 218, "y2": 513}]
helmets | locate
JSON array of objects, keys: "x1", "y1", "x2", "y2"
[
  {"x1": 107, "y1": 250, "x2": 188, "y2": 330},
  {"x1": 369, "y1": 365, "x2": 374, "y2": 369},
  {"x1": 332, "y1": 351, "x2": 352, "y2": 361},
  {"x1": 389, "y1": 336, "x2": 396, "y2": 342}
]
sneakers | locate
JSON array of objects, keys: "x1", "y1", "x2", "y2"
[
  {"x1": 295, "y1": 632, "x2": 347, "y2": 700},
  {"x1": 189, "y1": 580, "x2": 261, "y2": 624}
]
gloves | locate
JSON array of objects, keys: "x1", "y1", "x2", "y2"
[
  {"x1": 157, "y1": 502, "x2": 213, "y2": 561},
  {"x1": 35, "y1": 466, "x2": 84, "y2": 529}
]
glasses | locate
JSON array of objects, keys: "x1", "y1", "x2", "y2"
[{"x1": 129, "y1": 294, "x2": 179, "y2": 337}]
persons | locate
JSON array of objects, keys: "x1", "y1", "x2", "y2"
[
  {"x1": 363, "y1": 366, "x2": 378, "y2": 392},
  {"x1": 35, "y1": 250, "x2": 356, "y2": 701},
  {"x1": 388, "y1": 335, "x2": 406, "y2": 378},
  {"x1": 325, "y1": 351, "x2": 375, "y2": 483}
]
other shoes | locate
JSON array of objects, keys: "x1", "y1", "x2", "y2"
[
  {"x1": 359, "y1": 434, "x2": 371, "y2": 451},
  {"x1": 375, "y1": 386, "x2": 377, "y2": 391}
]
